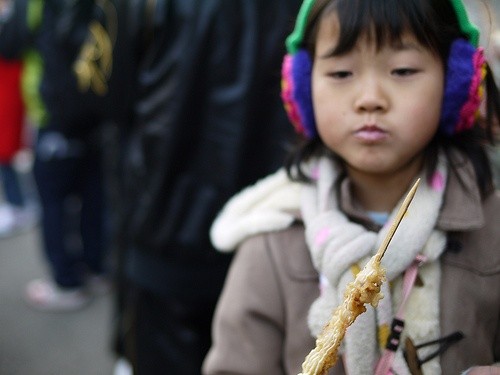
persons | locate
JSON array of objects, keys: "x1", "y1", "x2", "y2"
[
  {"x1": 199, "y1": 0, "x2": 500, "y2": 375},
  {"x1": 0, "y1": 0, "x2": 303, "y2": 375}
]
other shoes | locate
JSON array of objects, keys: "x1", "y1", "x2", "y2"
[{"x1": 22, "y1": 278, "x2": 90, "y2": 315}]
